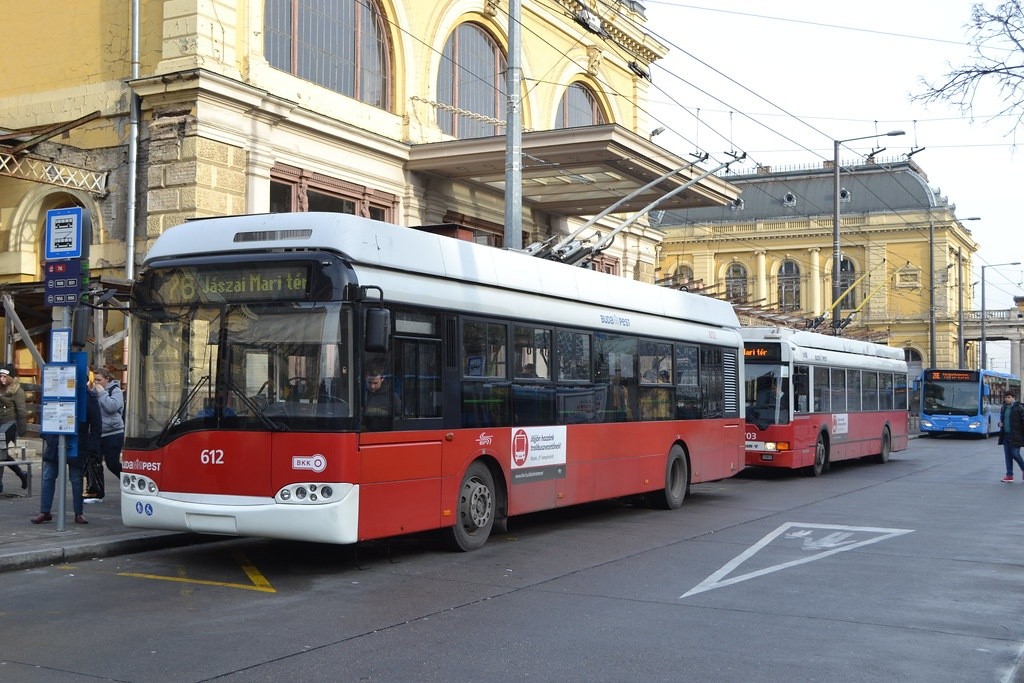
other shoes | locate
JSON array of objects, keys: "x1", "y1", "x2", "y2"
[
  {"x1": 82, "y1": 493, "x2": 100, "y2": 498},
  {"x1": 75, "y1": 513, "x2": 88, "y2": 524},
  {"x1": 23, "y1": 473, "x2": 32, "y2": 489},
  {"x1": 1022, "y1": 472, "x2": 1024, "y2": 480},
  {"x1": 31, "y1": 513, "x2": 53, "y2": 522},
  {"x1": 1001, "y1": 476, "x2": 1014, "y2": 481}
]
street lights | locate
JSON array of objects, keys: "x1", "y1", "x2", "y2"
[
  {"x1": 928, "y1": 217, "x2": 981, "y2": 368},
  {"x1": 980, "y1": 262, "x2": 1022, "y2": 370},
  {"x1": 831, "y1": 130, "x2": 907, "y2": 338}
]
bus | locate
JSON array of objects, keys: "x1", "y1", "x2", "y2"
[
  {"x1": 70, "y1": 151, "x2": 747, "y2": 553},
  {"x1": 914, "y1": 366, "x2": 1021, "y2": 439},
  {"x1": 735, "y1": 258, "x2": 911, "y2": 478}
]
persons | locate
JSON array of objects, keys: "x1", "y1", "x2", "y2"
[
  {"x1": 365, "y1": 367, "x2": 402, "y2": 414},
  {"x1": 998, "y1": 391, "x2": 1024, "y2": 482},
  {"x1": 766, "y1": 382, "x2": 787, "y2": 409},
  {"x1": 522, "y1": 364, "x2": 534, "y2": 377},
  {"x1": 84, "y1": 367, "x2": 124, "y2": 504},
  {"x1": 31, "y1": 377, "x2": 103, "y2": 525},
  {"x1": 0, "y1": 363, "x2": 32, "y2": 494},
  {"x1": 610, "y1": 370, "x2": 672, "y2": 418}
]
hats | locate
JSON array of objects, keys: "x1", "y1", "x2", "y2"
[{"x1": 0, "y1": 364, "x2": 15, "y2": 378}]
